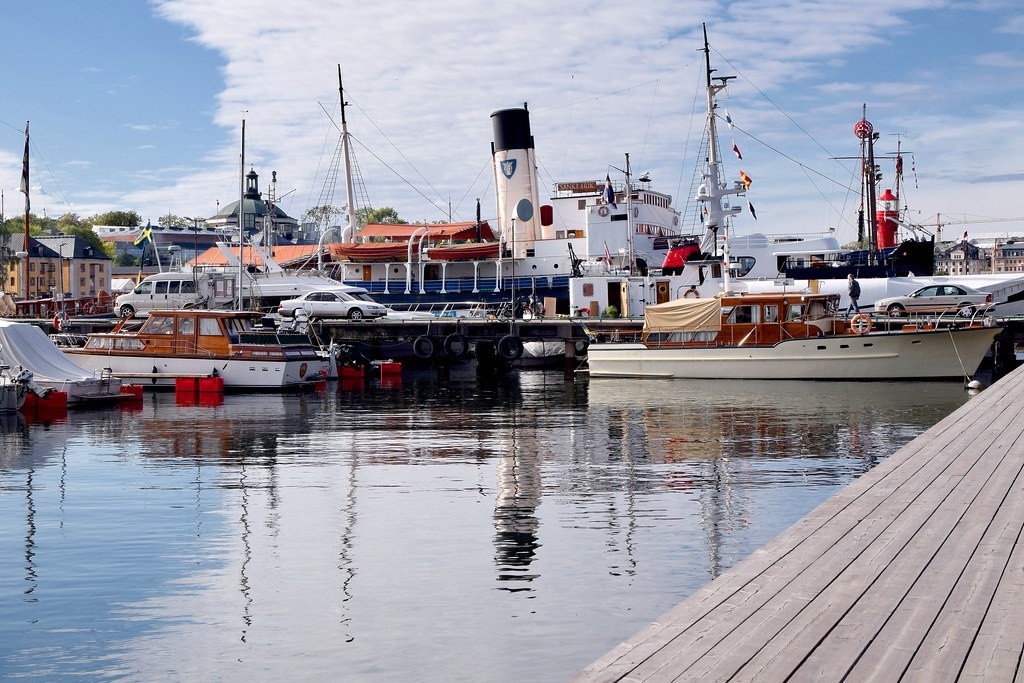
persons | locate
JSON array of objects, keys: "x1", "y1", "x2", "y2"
[{"x1": 843, "y1": 274, "x2": 861, "y2": 318}]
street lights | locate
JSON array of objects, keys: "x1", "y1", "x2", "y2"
[{"x1": 58, "y1": 242, "x2": 66, "y2": 322}]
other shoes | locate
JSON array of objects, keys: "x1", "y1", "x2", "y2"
[{"x1": 843, "y1": 314, "x2": 848, "y2": 317}]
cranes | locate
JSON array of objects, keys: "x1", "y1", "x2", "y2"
[{"x1": 911, "y1": 212, "x2": 1024, "y2": 244}]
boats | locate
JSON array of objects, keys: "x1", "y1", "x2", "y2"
[{"x1": 0, "y1": 23, "x2": 1024, "y2": 424}]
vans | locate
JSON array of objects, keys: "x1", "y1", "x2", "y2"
[{"x1": 113, "y1": 274, "x2": 213, "y2": 321}]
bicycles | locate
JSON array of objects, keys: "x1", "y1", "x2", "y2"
[{"x1": 495, "y1": 297, "x2": 533, "y2": 323}]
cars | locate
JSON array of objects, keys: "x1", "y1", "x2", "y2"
[
  {"x1": 277, "y1": 291, "x2": 387, "y2": 321},
  {"x1": 874, "y1": 284, "x2": 996, "y2": 318}
]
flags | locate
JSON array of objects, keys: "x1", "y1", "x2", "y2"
[
  {"x1": 603, "y1": 174, "x2": 618, "y2": 210},
  {"x1": 18, "y1": 123, "x2": 31, "y2": 211},
  {"x1": 963, "y1": 231, "x2": 968, "y2": 238},
  {"x1": 133, "y1": 222, "x2": 153, "y2": 250}
]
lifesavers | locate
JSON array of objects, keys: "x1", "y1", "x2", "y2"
[
  {"x1": 850, "y1": 313, "x2": 874, "y2": 336},
  {"x1": 444, "y1": 333, "x2": 468, "y2": 358},
  {"x1": 497, "y1": 334, "x2": 524, "y2": 361},
  {"x1": 53, "y1": 312, "x2": 72, "y2": 331},
  {"x1": 683, "y1": 289, "x2": 700, "y2": 299},
  {"x1": 411, "y1": 334, "x2": 434, "y2": 360}
]
